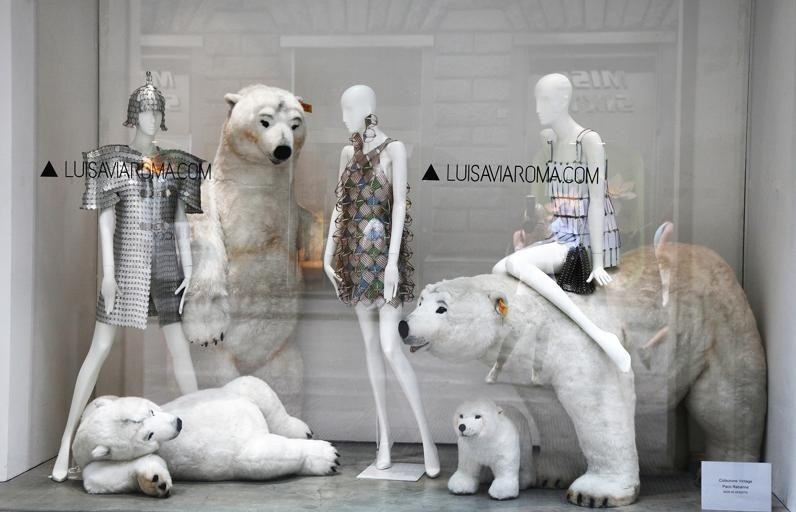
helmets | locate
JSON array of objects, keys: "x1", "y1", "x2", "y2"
[{"x1": 123, "y1": 71, "x2": 169, "y2": 131}]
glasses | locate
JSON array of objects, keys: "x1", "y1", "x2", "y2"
[{"x1": 363, "y1": 113, "x2": 378, "y2": 143}]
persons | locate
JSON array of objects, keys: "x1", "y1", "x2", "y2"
[
  {"x1": 492, "y1": 74, "x2": 631, "y2": 373},
  {"x1": 487, "y1": 203, "x2": 549, "y2": 385},
  {"x1": 52, "y1": 71, "x2": 203, "y2": 483},
  {"x1": 324, "y1": 84, "x2": 441, "y2": 478},
  {"x1": 637, "y1": 210, "x2": 672, "y2": 368}
]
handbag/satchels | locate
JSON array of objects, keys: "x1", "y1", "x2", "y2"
[{"x1": 558, "y1": 245, "x2": 596, "y2": 295}]
